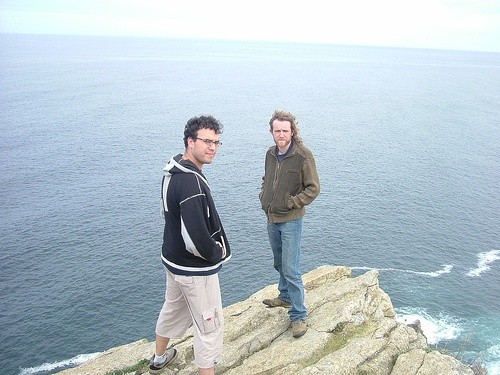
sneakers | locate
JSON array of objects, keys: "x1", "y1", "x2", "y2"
[
  {"x1": 149, "y1": 347, "x2": 177, "y2": 373},
  {"x1": 263, "y1": 297, "x2": 292, "y2": 307},
  {"x1": 292, "y1": 316, "x2": 307, "y2": 338}
]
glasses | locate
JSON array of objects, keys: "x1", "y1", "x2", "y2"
[{"x1": 193, "y1": 137, "x2": 223, "y2": 146}]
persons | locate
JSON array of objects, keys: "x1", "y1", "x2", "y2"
[
  {"x1": 149, "y1": 116, "x2": 232, "y2": 375},
  {"x1": 259, "y1": 111, "x2": 320, "y2": 338}
]
